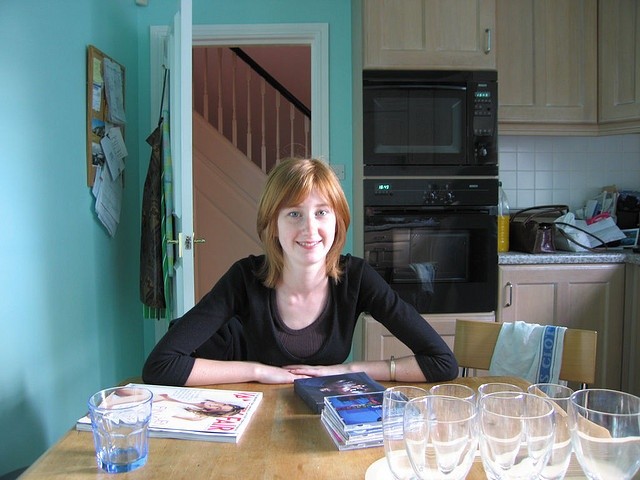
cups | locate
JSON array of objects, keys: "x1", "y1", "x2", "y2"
[{"x1": 89, "y1": 387, "x2": 153, "y2": 472}]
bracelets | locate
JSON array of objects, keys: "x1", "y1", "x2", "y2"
[{"x1": 390, "y1": 355, "x2": 396, "y2": 382}]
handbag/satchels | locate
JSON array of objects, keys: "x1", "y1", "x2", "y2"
[{"x1": 509, "y1": 219, "x2": 557, "y2": 254}]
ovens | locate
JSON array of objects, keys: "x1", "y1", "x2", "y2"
[{"x1": 365, "y1": 180, "x2": 497, "y2": 312}]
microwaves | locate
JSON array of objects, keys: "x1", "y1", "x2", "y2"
[{"x1": 362, "y1": 70, "x2": 499, "y2": 175}]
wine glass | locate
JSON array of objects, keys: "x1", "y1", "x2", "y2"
[
  {"x1": 526, "y1": 383, "x2": 575, "y2": 480},
  {"x1": 478, "y1": 392, "x2": 555, "y2": 480},
  {"x1": 407, "y1": 396, "x2": 477, "y2": 480},
  {"x1": 430, "y1": 384, "x2": 475, "y2": 456},
  {"x1": 382, "y1": 385, "x2": 428, "y2": 480},
  {"x1": 568, "y1": 389, "x2": 640, "y2": 480},
  {"x1": 476, "y1": 383, "x2": 524, "y2": 441}
]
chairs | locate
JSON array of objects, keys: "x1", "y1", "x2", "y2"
[{"x1": 455, "y1": 319, "x2": 599, "y2": 384}]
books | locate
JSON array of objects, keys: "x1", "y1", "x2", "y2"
[
  {"x1": 293, "y1": 372, "x2": 420, "y2": 451},
  {"x1": 76, "y1": 382, "x2": 263, "y2": 444}
]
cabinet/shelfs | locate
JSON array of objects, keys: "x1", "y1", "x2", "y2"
[
  {"x1": 499, "y1": 254, "x2": 626, "y2": 392},
  {"x1": 363, "y1": 0, "x2": 498, "y2": 70},
  {"x1": 623, "y1": 253, "x2": 640, "y2": 397},
  {"x1": 495, "y1": 0, "x2": 600, "y2": 136},
  {"x1": 598, "y1": 0, "x2": 640, "y2": 136},
  {"x1": 363, "y1": 311, "x2": 496, "y2": 377}
]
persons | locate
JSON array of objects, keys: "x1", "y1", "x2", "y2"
[
  {"x1": 90, "y1": 387, "x2": 242, "y2": 421},
  {"x1": 143, "y1": 157, "x2": 459, "y2": 384}
]
